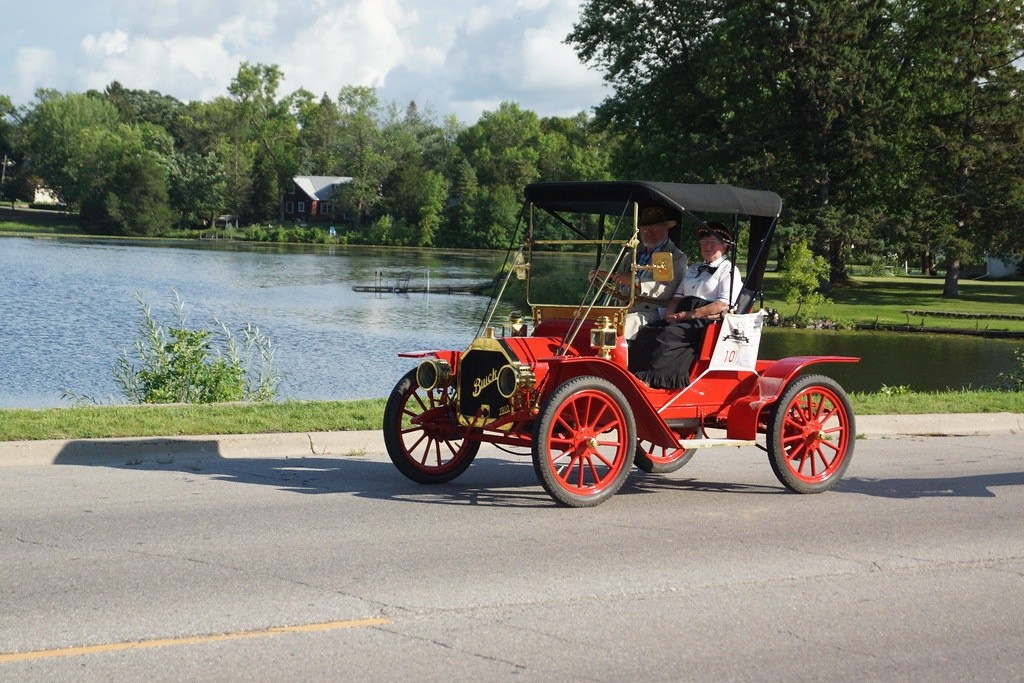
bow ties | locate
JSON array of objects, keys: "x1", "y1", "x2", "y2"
[{"x1": 694, "y1": 264, "x2": 719, "y2": 278}]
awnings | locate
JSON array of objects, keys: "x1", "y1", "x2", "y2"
[{"x1": 522, "y1": 178, "x2": 783, "y2": 216}]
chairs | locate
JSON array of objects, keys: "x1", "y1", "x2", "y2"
[{"x1": 689, "y1": 286, "x2": 759, "y2": 383}]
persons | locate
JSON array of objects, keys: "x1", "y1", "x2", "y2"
[
  {"x1": 628, "y1": 220, "x2": 744, "y2": 389},
  {"x1": 615, "y1": 208, "x2": 689, "y2": 348}
]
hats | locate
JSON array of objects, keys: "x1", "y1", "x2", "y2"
[
  {"x1": 697, "y1": 221, "x2": 732, "y2": 252},
  {"x1": 631, "y1": 207, "x2": 677, "y2": 229}
]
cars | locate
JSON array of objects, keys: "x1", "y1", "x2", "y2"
[{"x1": 382, "y1": 180, "x2": 861, "y2": 508}]
